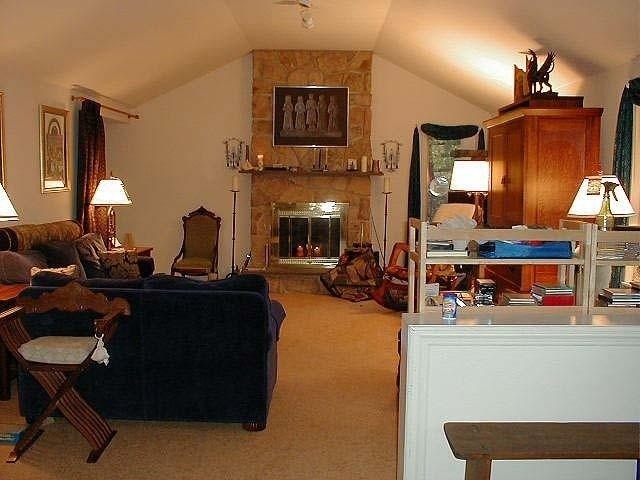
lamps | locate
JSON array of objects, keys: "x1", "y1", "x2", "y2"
[
  {"x1": 566, "y1": 173, "x2": 639, "y2": 227},
  {"x1": 450, "y1": 159, "x2": 492, "y2": 226},
  {"x1": 89, "y1": 178, "x2": 133, "y2": 253}
]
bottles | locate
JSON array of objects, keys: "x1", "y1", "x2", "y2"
[
  {"x1": 314, "y1": 246, "x2": 319, "y2": 256},
  {"x1": 297, "y1": 246, "x2": 302, "y2": 257},
  {"x1": 441, "y1": 293, "x2": 457, "y2": 321}
]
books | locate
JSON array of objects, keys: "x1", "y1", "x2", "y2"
[
  {"x1": 503, "y1": 281, "x2": 575, "y2": 306},
  {"x1": 427, "y1": 250, "x2": 468, "y2": 257},
  {"x1": 598, "y1": 288, "x2": 640, "y2": 307}
]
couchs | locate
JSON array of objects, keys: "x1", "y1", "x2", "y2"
[
  {"x1": 14, "y1": 269, "x2": 287, "y2": 430},
  {"x1": 0, "y1": 219, "x2": 141, "y2": 305}
]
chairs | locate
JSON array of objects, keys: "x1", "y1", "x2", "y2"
[
  {"x1": 170, "y1": 204, "x2": 222, "y2": 282},
  {"x1": 372, "y1": 203, "x2": 486, "y2": 317},
  {"x1": 0, "y1": 305, "x2": 130, "y2": 466}
]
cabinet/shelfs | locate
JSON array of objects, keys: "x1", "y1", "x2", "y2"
[
  {"x1": 481, "y1": 106, "x2": 606, "y2": 295},
  {"x1": 407, "y1": 218, "x2": 640, "y2": 480}
]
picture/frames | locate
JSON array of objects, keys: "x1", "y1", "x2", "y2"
[
  {"x1": 271, "y1": 85, "x2": 351, "y2": 150},
  {"x1": 38, "y1": 102, "x2": 72, "y2": 195}
]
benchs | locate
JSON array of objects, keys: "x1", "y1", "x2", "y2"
[{"x1": 441, "y1": 419, "x2": 640, "y2": 479}]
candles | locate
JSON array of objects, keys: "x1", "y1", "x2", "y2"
[
  {"x1": 232, "y1": 173, "x2": 239, "y2": 192},
  {"x1": 383, "y1": 173, "x2": 391, "y2": 192},
  {"x1": 314, "y1": 246, "x2": 321, "y2": 258},
  {"x1": 297, "y1": 245, "x2": 305, "y2": 256},
  {"x1": 306, "y1": 244, "x2": 312, "y2": 256}
]
